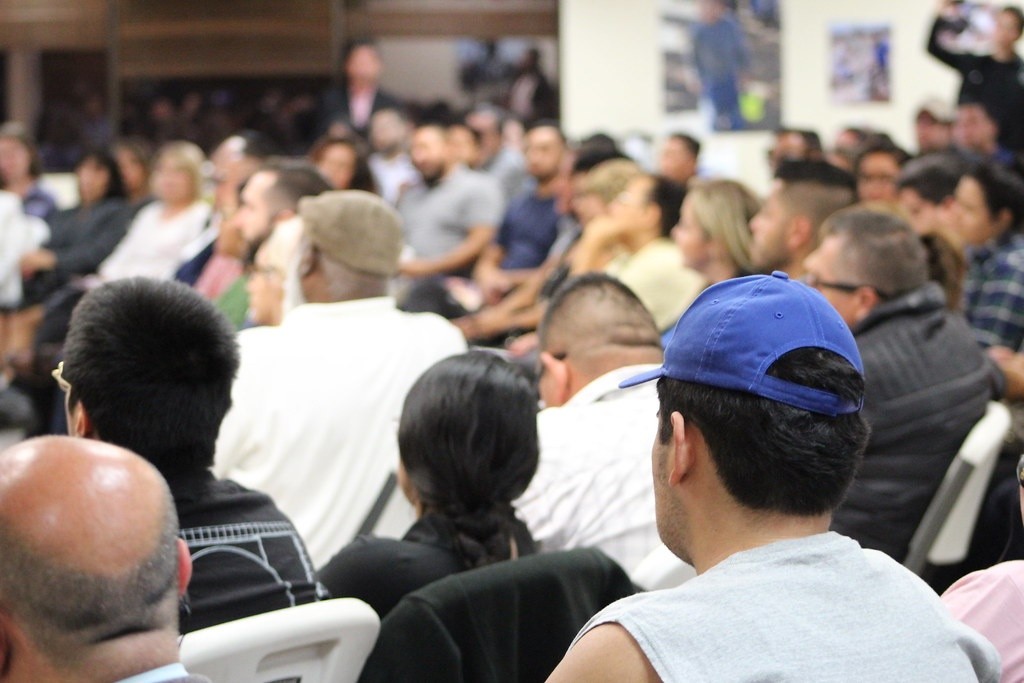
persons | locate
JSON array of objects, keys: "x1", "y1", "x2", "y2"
[
  {"x1": 0, "y1": 0, "x2": 1024, "y2": 683},
  {"x1": 691, "y1": 0, "x2": 750, "y2": 129}
]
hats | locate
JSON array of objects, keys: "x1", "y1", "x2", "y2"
[
  {"x1": 297, "y1": 189, "x2": 402, "y2": 275},
  {"x1": 618, "y1": 271, "x2": 865, "y2": 415}
]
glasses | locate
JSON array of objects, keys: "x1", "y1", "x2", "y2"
[
  {"x1": 802, "y1": 274, "x2": 890, "y2": 301},
  {"x1": 51, "y1": 360, "x2": 71, "y2": 388}
]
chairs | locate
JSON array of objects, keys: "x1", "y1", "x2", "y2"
[
  {"x1": 179, "y1": 548, "x2": 636, "y2": 683},
  {"x1": 902, "y1": 401, "x2": 1014, "y2": 577}
]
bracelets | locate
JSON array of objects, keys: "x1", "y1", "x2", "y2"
[{"x1": 213, "y1": 230, "x2": 250, "y2": 259}]
